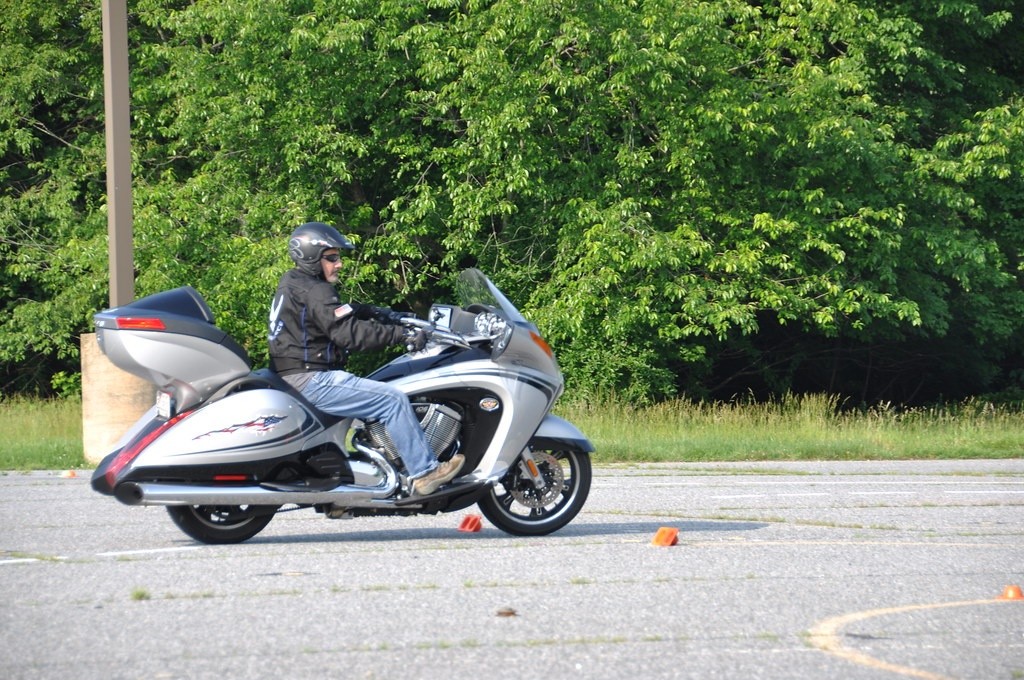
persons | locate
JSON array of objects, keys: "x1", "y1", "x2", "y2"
[{"x1": 267, "y1": 222, "x2": 465, "y2": 494}]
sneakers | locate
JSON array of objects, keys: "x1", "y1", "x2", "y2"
[{"x1": 415, "y1": 454, "x2": 465, "y2": 496}]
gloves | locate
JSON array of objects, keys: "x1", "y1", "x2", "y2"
[
  {"x1": 405, "y1": 328, "x2": 428, "y2": 352},
  {"x1": 388, "y1": 311, "x2": 417, "y2": 327}
]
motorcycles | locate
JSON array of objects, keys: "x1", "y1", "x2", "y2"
[{"x1": 90, "y1": 268, "x2": 597, "y2": 546}]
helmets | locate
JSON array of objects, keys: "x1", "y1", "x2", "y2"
[{"x1": 288, "y1": 222, "x2": 356, "y2": 282}]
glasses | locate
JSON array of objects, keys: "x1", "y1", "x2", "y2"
[{"x1": 321, "y1": 254, "x2": 340, "y2": 262}]
